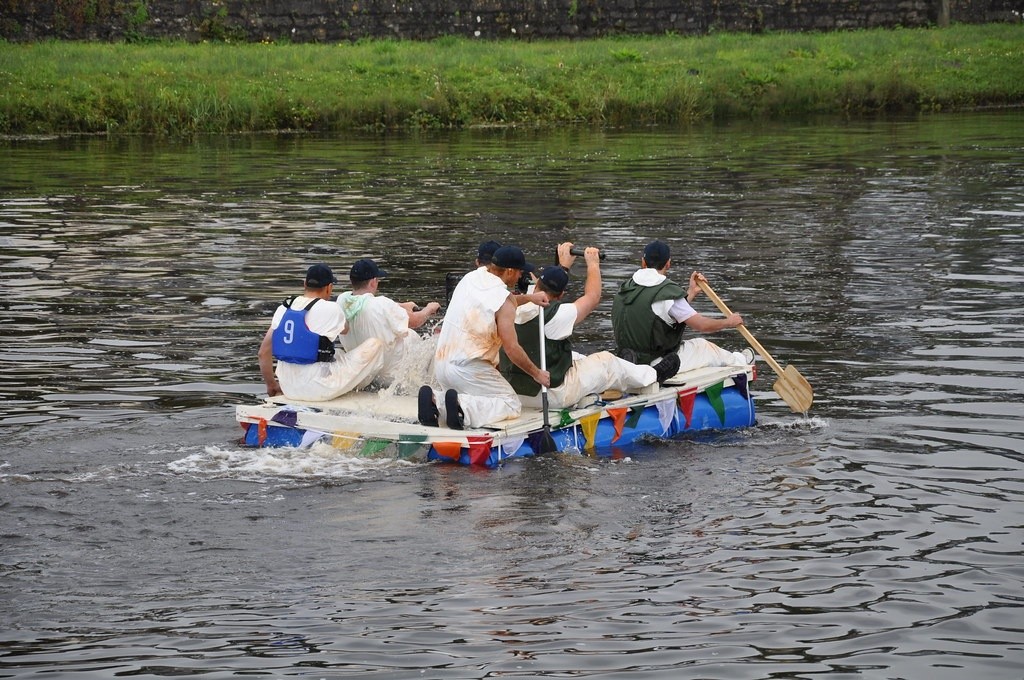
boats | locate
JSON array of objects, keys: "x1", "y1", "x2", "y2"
[{"x1": 235, "y1": 365, "x2": 758, "y2": 471}]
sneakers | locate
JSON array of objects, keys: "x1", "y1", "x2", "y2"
[
  {"x1": 619, "y1": 348, "x2": 637, "y2": 366},
  {"x1": 654, "y1": 352, "x2": 681, "y2": 389},
  {"x1": 741, "y1": 347, "x2": 755, "y2": 365}
]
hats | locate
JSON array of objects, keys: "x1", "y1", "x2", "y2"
[
  {"x1": 349, "y1": 258, "x2": 388, "y2": 281},
  {"x1": 491, "y1": 245, "x2": 535, "y2": 273},
  {"x1": 539, "y1": 267, "x2": 568, "y2": 292},
  {"x1": 306, "y1": 263, "x2": 338, "y2": 287},
  {"x1": 478, "y1": 240, "x2": 502, "y2": 260},
  {"x1": 644, "y1": 242, "x2": 671, "y2": 261}
]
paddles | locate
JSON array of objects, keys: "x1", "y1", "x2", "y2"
[
  {"x1": 529, "y1": 304, "x2": 558, "y2": 456},
  {"x1": 693, "y1": 274, "x2": 814, "y2": 413}
]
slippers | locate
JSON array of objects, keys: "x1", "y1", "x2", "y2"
[
  {"x1": 445, "y1": 388, "x2": 465, "y2": 430},
  {"x1": 418, "y1": 386, "x2": 439, "y2": 427}
]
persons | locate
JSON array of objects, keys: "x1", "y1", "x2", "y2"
[
  {"x1": 498, "y1": 266, "x2": 680, "y2": 410},
  {"x1": 258, "y1": 264, "x2": 384, "y2": 402},
  {"x1": 417, "y1": 246, "x2": 551, "y2": 434},
  {"x1": 475, "y1": 241, "x2": 502, "y2": 268},
  {"x1": 333, "y1": 258, "x2": 442, "y2": 395},
  {"x1": 612, "y1": 240, "x2": 756, "y2": 380}
]
